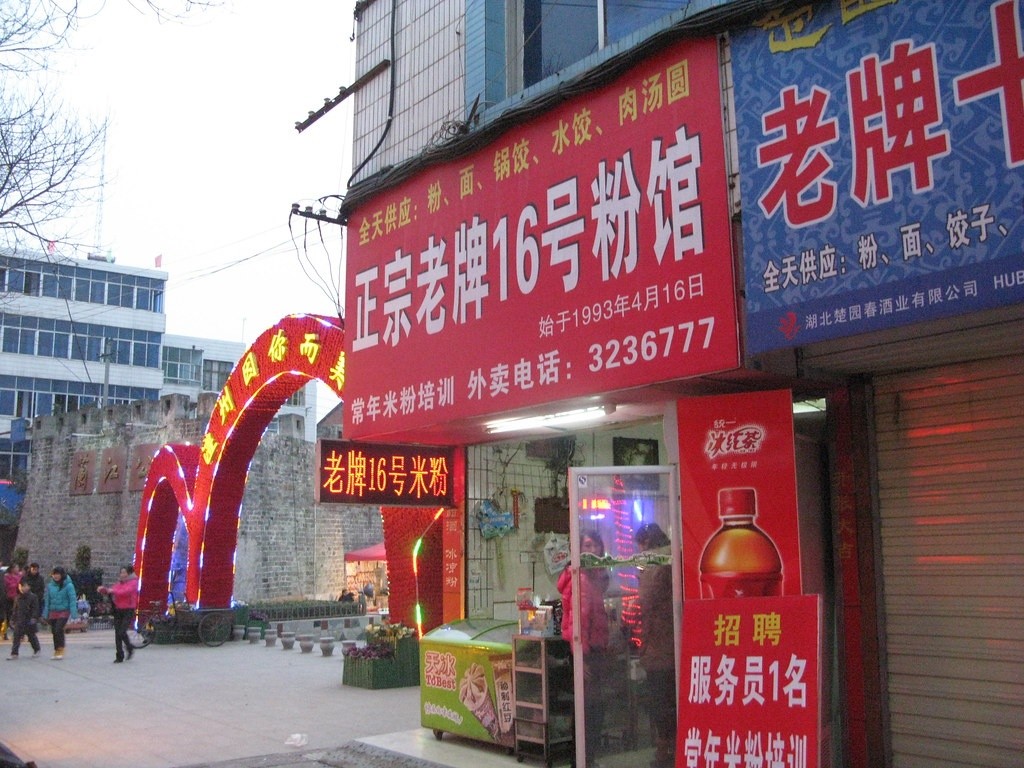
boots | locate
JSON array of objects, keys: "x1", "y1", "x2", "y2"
[
  {"x1": 127, "y1": 644, "x2": 133, "y2": 660},
  {"x1": 113, "y1": 651, "x2": 124, "y2": 663},
  {"x1": 650, "y1": 738, "x2": 676, "y2": 768}
]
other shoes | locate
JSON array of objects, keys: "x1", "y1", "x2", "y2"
[
  {"x1": 3, "y1": 636, "x2": 9, "y2": 640},
  {"x1": 33, "y1": 648, "x2": 41, "y2": 657},
  {"x1": 7, "y1": 654, "x2": 18, "y2": 660},
  {"x1": 570, "y1": 757, "x2": 601, "y2": 768}
]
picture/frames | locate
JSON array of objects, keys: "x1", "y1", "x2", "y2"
[{"x1": 613, "y1": 437, "x2": 660, "y2": 491}]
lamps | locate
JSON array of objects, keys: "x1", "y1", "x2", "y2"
[{"x1": 485, "y1": 401, "x2": 617, "y2": 435}]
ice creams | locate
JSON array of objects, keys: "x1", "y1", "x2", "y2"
[{"x1": 458, "y1": 662, "x2": 501, "y2": 745}]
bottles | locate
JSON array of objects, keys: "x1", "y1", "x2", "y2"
[
  {"x1": 515, "y1": 587, "x2": 534, "y2": 606},
  {"x1": 698, "y1": 486, "x2": 785, "y2": 604},
  {"x1": 519, "y1": 607, "x2": 536, "y2": 636}
]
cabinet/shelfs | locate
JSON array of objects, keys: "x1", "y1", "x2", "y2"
[{"x1": 512, "y1": 636, "x2": 634, "y2": 768}]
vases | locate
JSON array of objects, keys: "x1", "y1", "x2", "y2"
[
  {"x1": 234, "y1": 609, "x2": 270, "y2": 639},
  {"x1": 343, "y1": 639, "x2": 420, "y2": 689}
]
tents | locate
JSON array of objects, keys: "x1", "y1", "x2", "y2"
[{"x1": 345, "y1": 542, "x2": 386, "y2": 587}]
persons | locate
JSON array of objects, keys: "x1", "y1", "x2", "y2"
[
  {"x1": 97, "y1": 565, "x2": 138, "y2": 663},
  {"x1": 635, "y1": 523, "x2": 676, "y2": 768},
  {"x1": 41, "y1": 566, "x2": 77, "y2": 659},
  {"x1": 557, "y1": 528, "x2": 610, "y2": 768},
  {"x1": 0, "y1": 559, "x2": 44, "y2": 660},
  {"x1": 339, "y1": 589, "x2": 366, "y2": 603}
]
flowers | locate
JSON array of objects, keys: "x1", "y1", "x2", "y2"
[
  {"x1": 366, "y1": 622, "x2": 417, "y2": 642},
  {"x1": 343, "y1": 643, "x2": 394, "y2": 660},
  {"x1": 249, "y1": 610, "x2": 269, "y2": 621},
  {"x1": 234, "y1": 599, "x2": 249, "y2": 608}
]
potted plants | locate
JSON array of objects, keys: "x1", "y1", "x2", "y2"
[{"x1": 67, "y1": 544, "x2": 104, "y2": 615}]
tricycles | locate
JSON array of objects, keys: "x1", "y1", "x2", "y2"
[{"x1": 124, "y1": 591, "x2": 233, "y2": 649}]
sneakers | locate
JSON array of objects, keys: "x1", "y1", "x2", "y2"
[
  {"x1": 51, "y1": 650, "x2": 57, "y2": 659},
  {"x1": 57, "y1": 646, "x2": 64, "y2": 660}
]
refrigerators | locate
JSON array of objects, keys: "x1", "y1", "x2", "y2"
[{"x1": 420, "y1": 615, "x2": 516, "y2": 755}]
